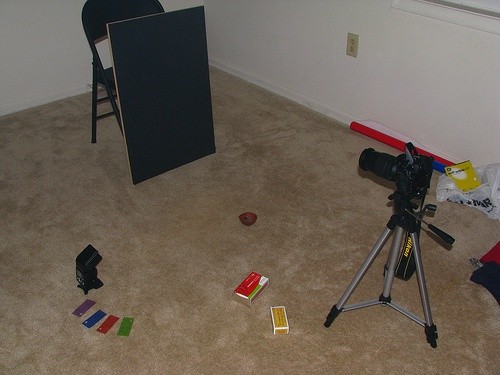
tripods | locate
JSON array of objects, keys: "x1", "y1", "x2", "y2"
[{"x1": 324, "y1": 192, "x2": 454, "y2": 348}]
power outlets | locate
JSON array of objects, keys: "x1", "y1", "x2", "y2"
[{"x1": 346, "y1": 33, "x2": 359, "y2": 58}]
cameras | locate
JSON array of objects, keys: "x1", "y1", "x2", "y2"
[{"x1": 359, "y1": 141, "x2": 433, "y2": 200}]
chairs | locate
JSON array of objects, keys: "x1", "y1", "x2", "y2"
[{"x1": 81, "y1": 0, "x2": 166, "y2": 145}]
